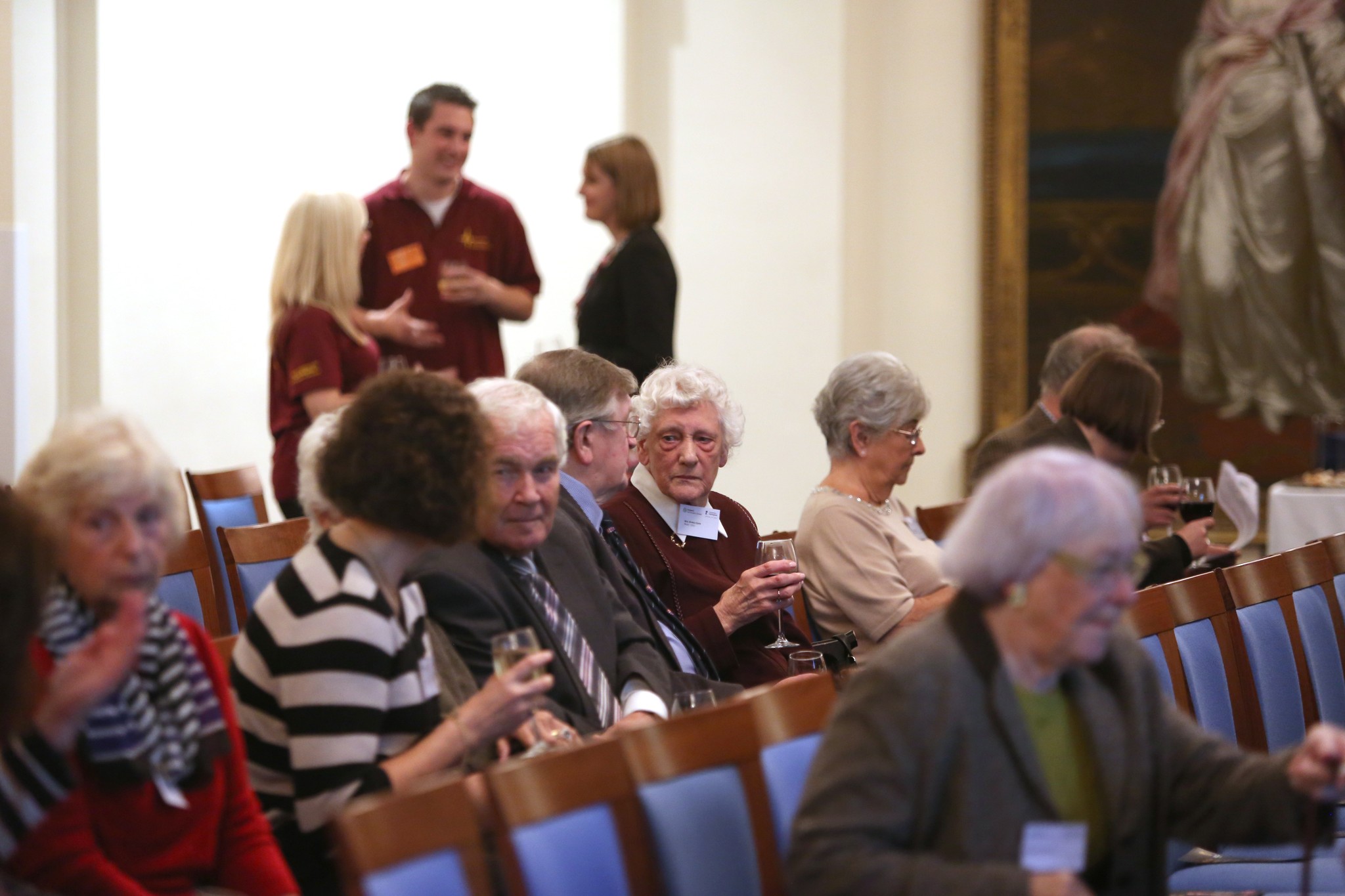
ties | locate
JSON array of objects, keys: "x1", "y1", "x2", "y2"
[
  {"x1": 509, "y1": 551, "x2": 623, "y2": 731},
  {"x1": 601, "y1": 509, "x2": 719, "y2": 683}
]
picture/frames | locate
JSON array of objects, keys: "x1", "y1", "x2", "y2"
[{"x1": 982, "y1": 0, "x2": 1345, "y2": 545}]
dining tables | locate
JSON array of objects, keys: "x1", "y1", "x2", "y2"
[{"x1": 1266, "y1": 479, "x2": 1345, "y2": 556}]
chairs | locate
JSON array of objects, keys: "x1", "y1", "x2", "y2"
[{"x1": 153, "y1": 467, "x2": 1345, "y2": 896}]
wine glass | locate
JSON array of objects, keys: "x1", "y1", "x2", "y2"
[
  {"x1": 1178, "y1": 476, "x2": 1217, "y2": 570},
  {"x1": 1146, "y1": 465, "x2": 1184, "y2": 537},
  {"x1": 755, "y1": 539, "x2": 801, "y2": 649},
  {"x1": 490, "y1": 626, "x2": 573, "y2": 755}
]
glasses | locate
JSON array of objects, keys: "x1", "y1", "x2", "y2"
[
  {"x1": 891, "y1": 427, "x2": 922, "y2": 444},
  {"x1": 1051, "y1": 549, "x2": 1150, "y2": 589},
  {"x1": 567, "y1": 415, "x2": 642, "y2": 438}
]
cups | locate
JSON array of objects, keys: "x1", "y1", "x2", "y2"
[
  {"x1": 435, "y1": 260, "x2": 470, "y2": 299},
  {"x1": 671, "y1": 689, "x2": 717, "y2": 715},
  {"x1": 788, "y1": 651, "x2": 827, "y2": 677}
]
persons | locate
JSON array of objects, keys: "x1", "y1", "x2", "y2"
[
  {"x1": 795, "y1": 351, "x2": 961, "y2": 657},
  {"x1": 600, "y1": 363, "x2": 816, "y2": 688},
  {"x1": 355, "y1": 84, "x2": 541, "y2": 386},
  {"x1": 1024, "y1": 348, "x2": 1232, "y2": 594},
  {"x1": 1143, "y1": 0, "x2": 1345, "y2": 435},
  {"x1": 400, "y1": 375, "x2": 670, "y2": 755},
  {"x1": 575, "y1": 135, "x2": 677, "y2": 396},
  {"x1": 0, "y1": 405, "x2": 302, "y2": 896},
  {"x1": 227, "y1": 368, "x2": 555, "y2": 896},
  {"x1": 270, "y1": 191, "x2": 380, "y2": 520},
  {"x1": 515, "y1": 348, "x2": 746, "y2": 710},
  {"x1": 296, "y1": 411, "x2": 496, "y2": 785},
  {"x1": 785, "y1": 443, "x2": 1345, "y2": 896},
  {"x1": 967, "y1": 324, "x2": 1182, "y2": 531}
]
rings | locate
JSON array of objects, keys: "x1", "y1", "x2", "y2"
[{"x1": 778, "y1": 588, "x2": 780, "y2": 598}]
